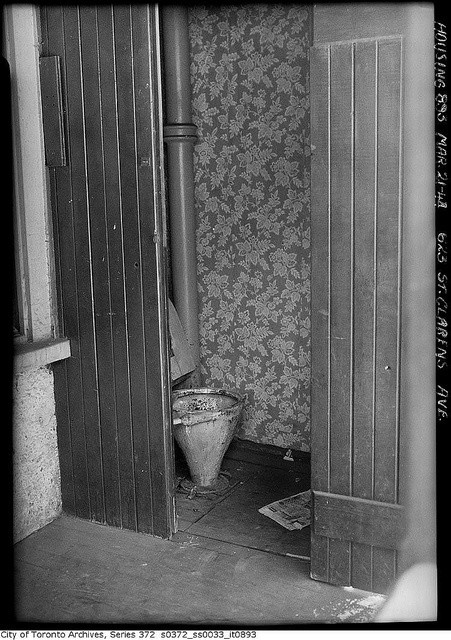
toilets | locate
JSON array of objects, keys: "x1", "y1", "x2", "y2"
[{"x1": 167, "y1": 295, "x2": 244, "y2": 495}]
